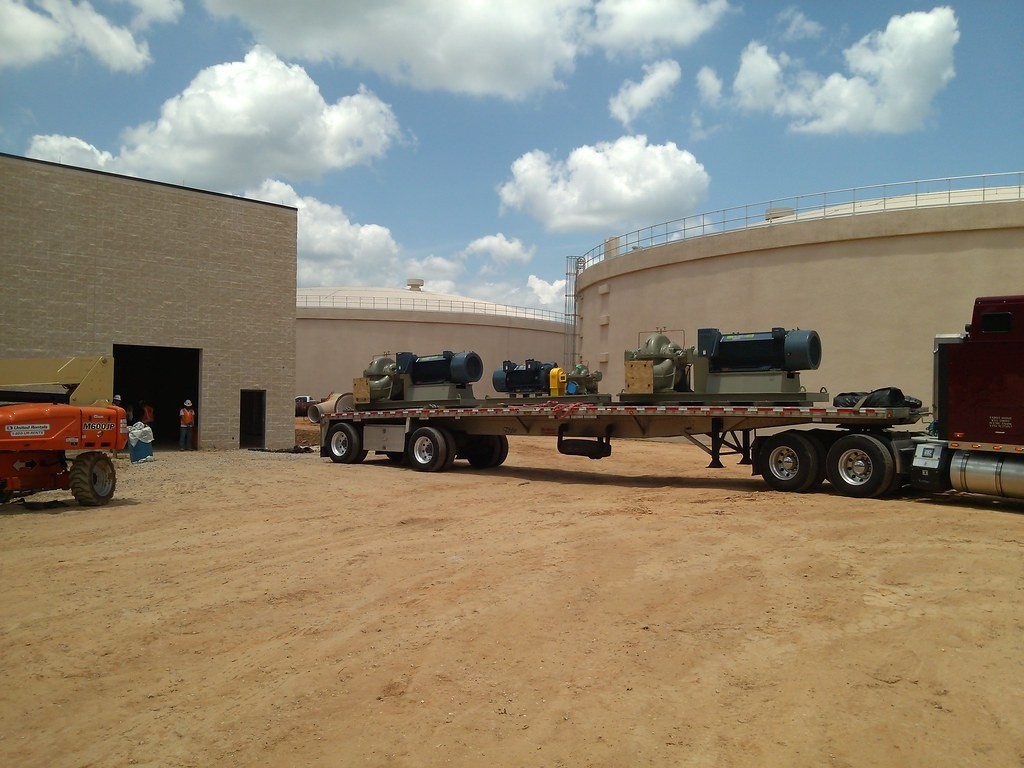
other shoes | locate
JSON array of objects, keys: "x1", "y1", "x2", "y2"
[
  {"x1": 186, "y1": 447, "x2": 193, "y2": 450},
  {"x1": 179, "y1": 447, "x2": 184, "y2": 451}
]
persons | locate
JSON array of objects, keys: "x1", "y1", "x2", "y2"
[
  {"x1": 179, "y1": 400, "x2": 195, "y2": 451},
  {"x1": 141, "y1": 400, "x2": 156, "y2": 446},
  {"x1": 112, "y1": 395, "x2": 121, "y2": 405}
]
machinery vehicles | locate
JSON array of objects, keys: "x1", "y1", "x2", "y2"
[{"x1": 1, "y1": 384, "x2": 127, "y2": 506}]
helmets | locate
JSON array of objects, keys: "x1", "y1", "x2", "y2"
[
  {"x1": 113, "y1": 395, "x2": 121, "y2": 400},
  {"x1": 139, "y1": 400, "x2": 146, "y2": 407},
  {"x1": 184, "y1": 400, "x2": 192, "y2": 406}
]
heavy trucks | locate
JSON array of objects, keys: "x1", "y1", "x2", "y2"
[{"x1": 309, "y1": 295, "x2": 1024, "y2": 498}]
